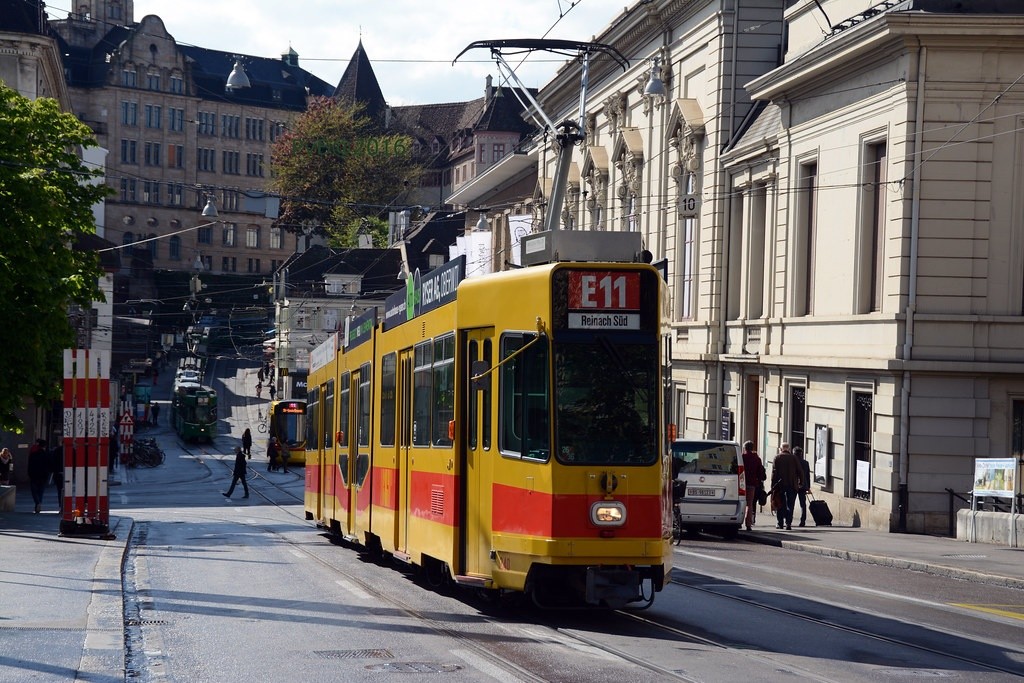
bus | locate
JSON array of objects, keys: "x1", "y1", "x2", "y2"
[
  {"x1": 268, "y1": 398, "x2": 308, "y2": 465},
  {"x1": 170, "y1": 356, "x2": 218, "y2": 445},
  {"x1": 302, "y1": 38, "x2": 675, "y2": 614}
]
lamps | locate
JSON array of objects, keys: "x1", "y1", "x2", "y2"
[
  {"x1": 644, "y1": 59, "x2": 667, "y2": 96},
  {"x1": 202, "y1": 192, "x2": 220, "y2": 218},
  {"x1": 225, "y1": 56, "x2": 253, "y2": 89}
]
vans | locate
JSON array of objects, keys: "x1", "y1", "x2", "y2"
[{"x1": 671, "y1": 437, "x2": 748, "y2": 541}]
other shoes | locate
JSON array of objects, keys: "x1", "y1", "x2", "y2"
[
  {"x1": 776, "y1": 522, "x2": 783, "y2": 529},
  {"x1": 272, "y1": 470, "x2": 277, "y2": 472},
  {"x1": 284, "y1": 471, "x2": 289, "y2": 473},
  {"x1": 799, "y1": 524, "x2": 805, "y2": 526},
  {"x1": 786, "y1": 525, "x2": 791, "y2": 530},
  {"x1": 747, "y1": 527, "x2": 752, "y2": 531},
  {"x1": 242, "y1": 496, "x2": 249, "y2": 499},
  {"x1": 223, "y1": 493, "x2": 230, "y2": 498},
  {"x1": 36, "y1": 504, "x2": 41, "y2": 513},
  {"x1": 267, "y1": 469, "x2": 272, "y2": 472},
  {"x1": 277, "y1": 466, "x2": 279, "y2": 471}
]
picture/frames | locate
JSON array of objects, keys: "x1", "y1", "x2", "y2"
[{"x1": 813, "y1": 423, "x2": 829, "y2": 488}]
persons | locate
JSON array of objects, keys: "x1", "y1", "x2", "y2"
[
  {"x1": 109, "y1": 418, "x2": 120, "y2": 474},
  {"x1": 0, "y1": 448, "x2": 14, "y2": 486},
  {"x1": 27, "y1": 438, "x2": 51, "y2": 514},
  {"x1": 255, "y1": 361, "x2": 276, "y2": 403},
  {"x1": 276, "y1": 437, "x2": 291, "y2": 473},
  {"x1": 152, "y1": 368, "x2": 160, "y2": 386},
  {"x1": 267, "y1": 437, "x2": 279, "y2": 471},
  {"x1": 789, "y1": 446, "x2": 812, "y2": 527},
  {"x1": 50, "y1": 438, "x2": 65, "y2": 515},
  {"x1": 150, "y1": 402, "x2": 160, "y2": 425},
  {"x1": 223, "y1": 446, "x2": 249, "y2": 498},
  {"x1": 740, "y1": 440, "x2": 761, "y2": 530},
  {"x1": 242, "y1": 428, "x2": 252, "y2": 459},
  {"x1": 750, "y1": 450, "x2": 767, "y2": 524},
  {"x1": 770, "y1": 442, "x2": 804, "y2": 530}
]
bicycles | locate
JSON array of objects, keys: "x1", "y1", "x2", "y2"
[{"x1": 128, "y1": 436, "x2": 166, "y2": 469}]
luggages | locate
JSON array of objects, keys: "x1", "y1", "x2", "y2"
[{"x1": 806, "y1": 492, "x2": 834, "y2": 526}]
